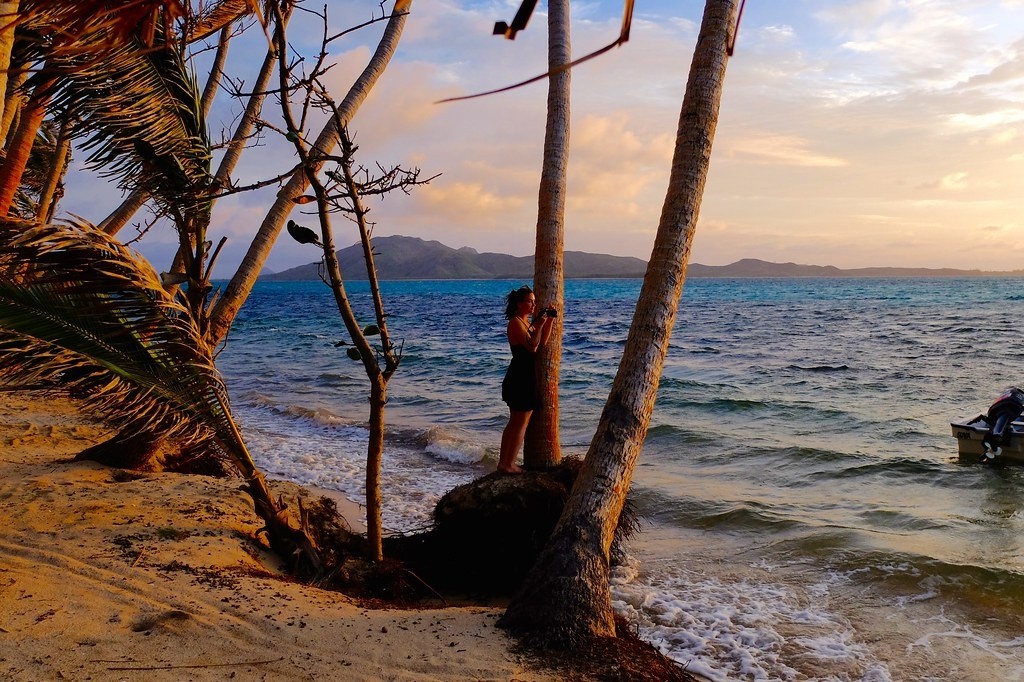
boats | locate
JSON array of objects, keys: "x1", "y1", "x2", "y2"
[{"x1": 950, "y1": 381, "x2": 1024, "y2": 472}]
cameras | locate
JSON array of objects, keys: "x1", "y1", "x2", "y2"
[{"x1": 540, "y1": 307, "x2": 558, "y2": 318}]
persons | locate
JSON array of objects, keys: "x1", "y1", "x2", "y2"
[{"x1": 497, "y1": 284, "x2": 555, "y2": 474}]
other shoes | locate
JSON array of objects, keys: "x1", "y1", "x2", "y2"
[{"x1": 497, "y1": 464, "x2": 526, "y2": 476}]
